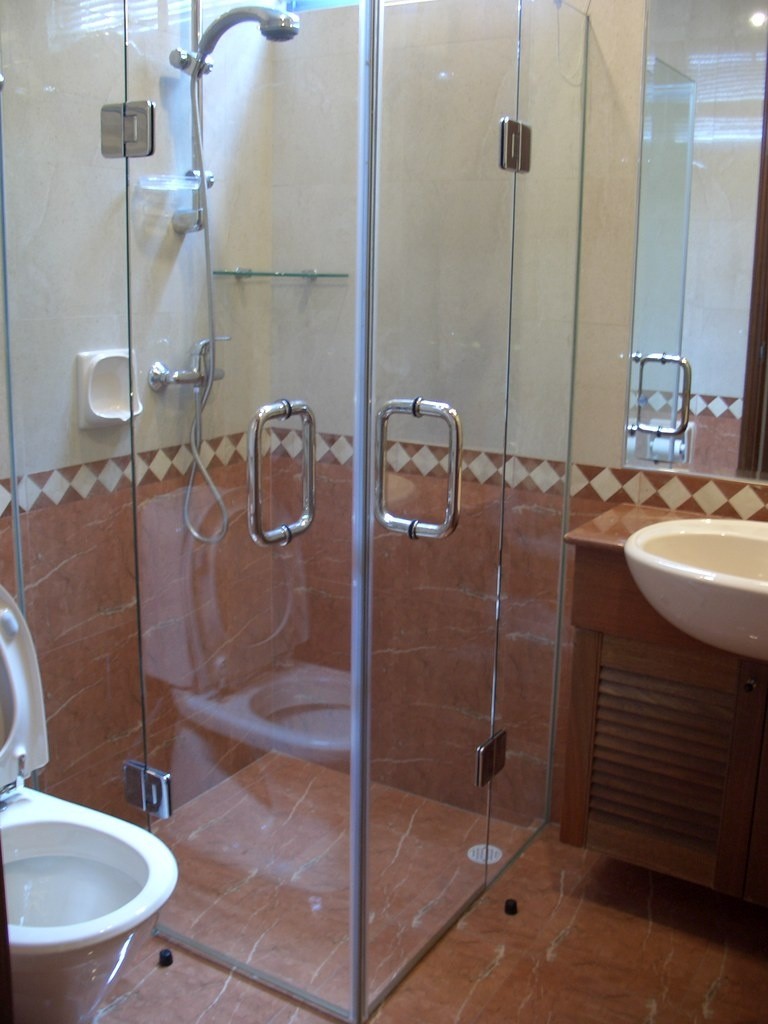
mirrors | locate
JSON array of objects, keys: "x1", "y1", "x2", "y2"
[{"x1": 620, "y1": 1, "x2": 768, "y2": 484}]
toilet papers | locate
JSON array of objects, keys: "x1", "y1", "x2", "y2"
[{"x1": 652, "y1": 437, "x2": 684, "y2": 461}]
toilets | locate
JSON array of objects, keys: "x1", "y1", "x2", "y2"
[
  {"x1": 136, "y1": 482, "x2": 368, "y2": 895},
  {"x1": 0, "y1": 579, "x2": 179, "y2": 1024}
]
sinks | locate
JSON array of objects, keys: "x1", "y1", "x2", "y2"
[{"x1": 624, "y1": 518, "x2": 768, "y2": 658}]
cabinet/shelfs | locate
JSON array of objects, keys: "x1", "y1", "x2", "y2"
[{"x1": 551, "y1": 502, "x2": 767, "y2": 906}]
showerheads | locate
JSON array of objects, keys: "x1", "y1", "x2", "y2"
[{"x1": 196, "y1": 5, "x2": 301, "y2": 55}]
